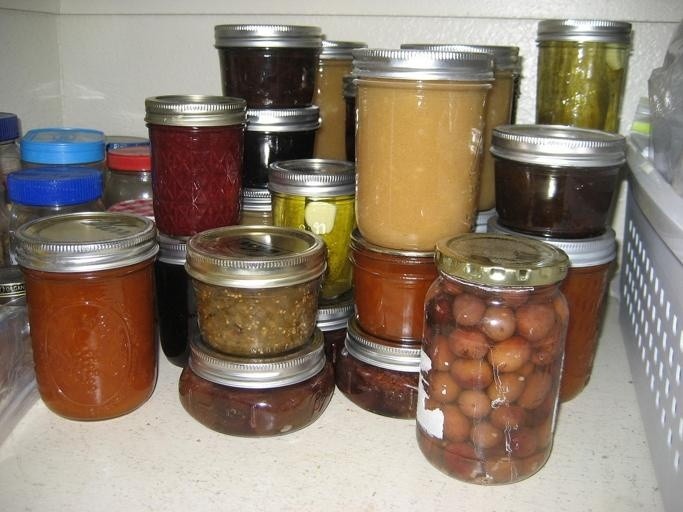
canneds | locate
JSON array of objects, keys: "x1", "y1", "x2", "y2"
[{"x1": 0, "y1": 20, "x2": 633, "y2": 486}]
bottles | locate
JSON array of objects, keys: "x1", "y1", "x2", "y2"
[{"x1": 0, "y1": 15, "x2": 637, "y2": 488}]
